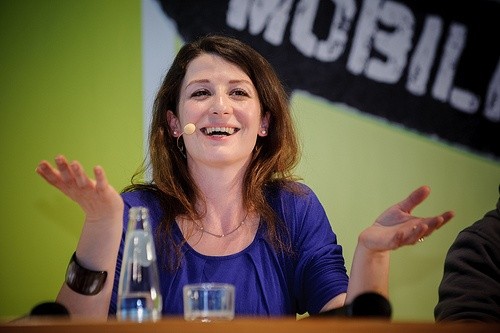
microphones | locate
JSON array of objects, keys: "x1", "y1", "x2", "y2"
[
  {"x1": 308, "y1": 291, "x2": 393, "y2": 321},
  {"x1": 177, "y1": 123, "x2": 196, "y2": 152}
]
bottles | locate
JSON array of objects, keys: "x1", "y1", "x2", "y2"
[{"x1": 116, "y1": 207, "x2": 162, "y2": 321}]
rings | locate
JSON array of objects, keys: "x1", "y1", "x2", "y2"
[{"x1": 418, "y1": 238, "x2": 424, "y2": 244}]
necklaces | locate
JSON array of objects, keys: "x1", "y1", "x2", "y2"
[{"x1": 190, "y1": 207, "x2": 250, "y2": 239}]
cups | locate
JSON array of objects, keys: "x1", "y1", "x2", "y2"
[{"x1": 182, "y1": 283, "x2": 236, "y2": 319}]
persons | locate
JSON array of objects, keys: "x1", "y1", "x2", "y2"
[
  {"x1": 433, "y1": 184, "x2": 500, "y2": 321},
  {"x1": 37, "y1": 38, "x2": 455, "y2": 318}
]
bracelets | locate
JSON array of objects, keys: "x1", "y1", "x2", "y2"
[{"x1": 66, "y1": 251, "x2": 107, "y2": 297}]
